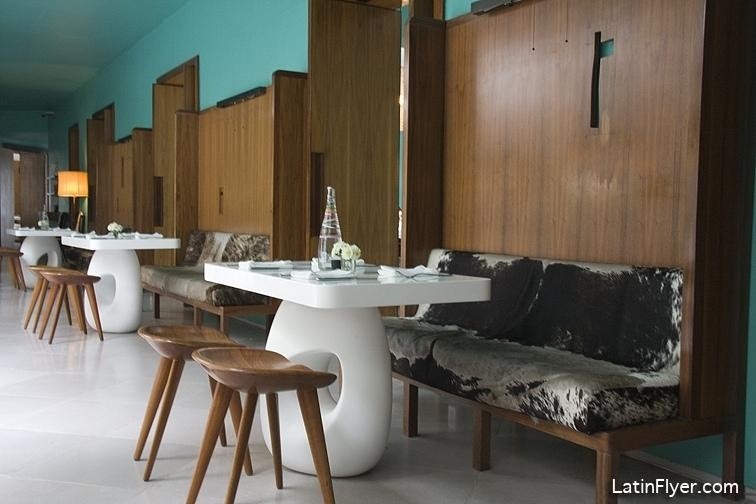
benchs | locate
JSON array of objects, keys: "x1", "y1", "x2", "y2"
[
  {"x1": 380, "y1": 247, "x2": 686, "y2": 504},
  {"x1": 136, "y1": 228, "x2": 272, "y2": 337}
]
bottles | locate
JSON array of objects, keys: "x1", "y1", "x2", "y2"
[{"x1": 318, "y1": 186, "x2": 342, "y2": 270}]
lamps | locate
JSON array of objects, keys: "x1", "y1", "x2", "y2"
[{"x1": 58, "y1": 171, "x2": 88, "y2": 230}]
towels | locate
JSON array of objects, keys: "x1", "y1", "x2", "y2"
[
  {"x1": 136, "y1": 231, "x2": 163, "y2": 238},
  {"x1": 239, "y1": 259, "x2": 292, "y2": 272},
  {"x1": 311, "y1": 258, "x2": 352, "y2": 277},
  {"x1": 118, "y1": 232, "x2": 135, "y2": 239},
  {"x1": 19, "y1": 226, "x2": 28, "y2": 229},
  {"x1": 72, "y1": 230, "x2": 95, "y2": 237},
  {"x1": 357, "y1": 259, "x2": 364, "y2": 265},
  {"x1": 27, "y1": 226, "x2": 35, "y2": 229},
  {"x1": 86, "y1": 232, "x2": 114, "y2": 240},
  {"x1": 377, "y1": 265, "x2": 441, "y2": 277},
  {"x1": 53, "y1": 227, "x2": 71, "y2": 232}
]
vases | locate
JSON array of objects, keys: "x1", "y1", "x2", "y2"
[{"x1": 339, "y1": 259, "x2": 356, "y2": 271}]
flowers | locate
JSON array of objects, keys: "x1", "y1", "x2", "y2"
[
  {"x1": 108, "y1": 222, "x2": 123, "y2": 239},
  {"x1": 331, "y1": 240, "x2": 361, "y2": 260}
]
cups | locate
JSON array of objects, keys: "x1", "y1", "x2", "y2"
[
  {"x1": 331, "y1": 260, "x2": 341, "y2": 270},
  {"x1": 280, "y1": 262, "x2": 292, "y2": 276}
]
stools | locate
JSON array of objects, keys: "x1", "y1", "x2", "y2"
[
  {"x1": 186, "y1": 346, "x2": 338, "y2": 504},
  {"x1": 134, "y1": 317, "x2": 253, "y2": 481},
  {"x1": 0, "y1": 247, "x2": 27, "y2": 292},
  {"x1": 24, "y1": 263, "x2": 82, "y2": 334},
  {"x1": 38, "y1": 270, "x2": 104, "y2": 344}
]
